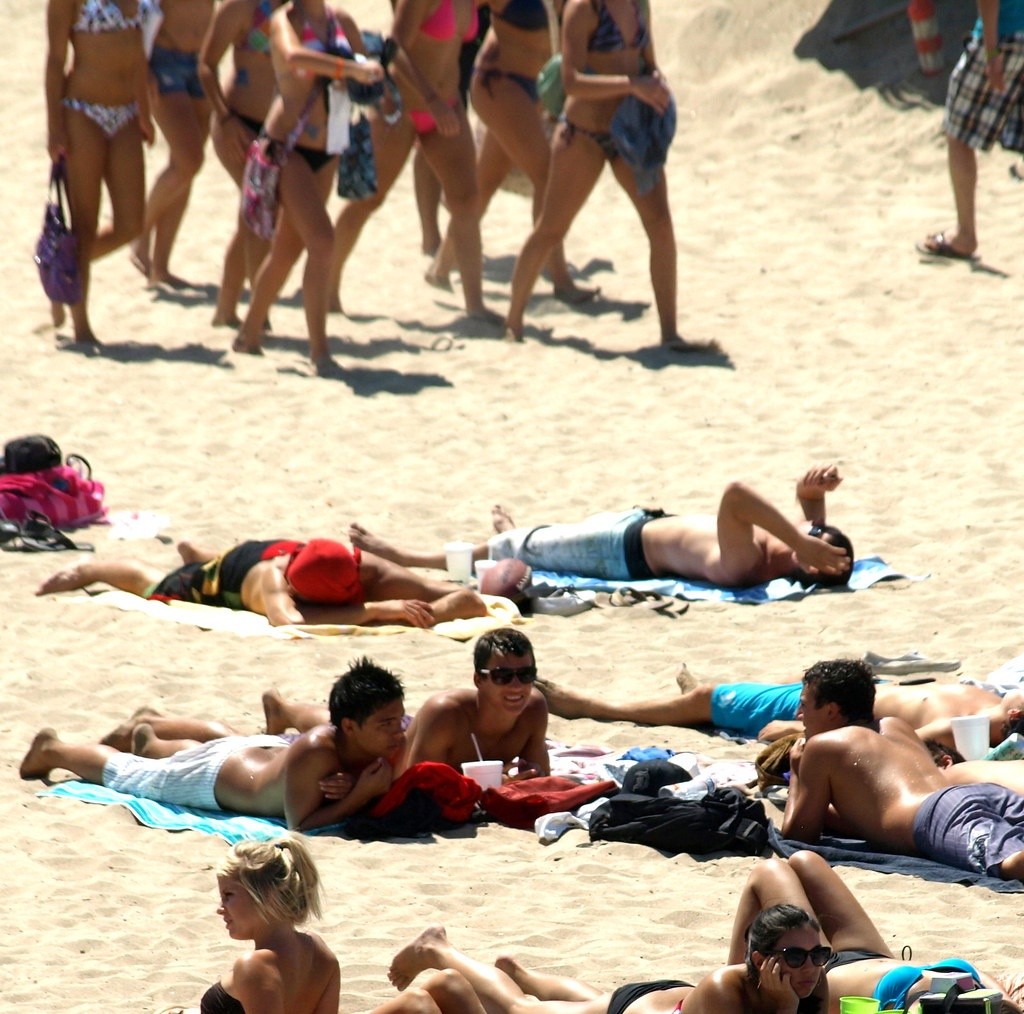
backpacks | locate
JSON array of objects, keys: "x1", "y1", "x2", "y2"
[
  {"x1": 619, "y1": 761, "x2": 692, "y2": 799},
  {"x1": 589, "y1": 787, "x2": 769, "y2": 853}
]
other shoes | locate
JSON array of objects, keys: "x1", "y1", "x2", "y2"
[{"x1": 535, "y1": 587, "x2": 597, "y2": 618}]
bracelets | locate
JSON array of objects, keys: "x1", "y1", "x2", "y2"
[
  {"x1": 334, "y1": 56, "x2": 342, "y2": 78},
  {"x1": 984, "y1": 48, "x2": 1000, "y2": 57}
]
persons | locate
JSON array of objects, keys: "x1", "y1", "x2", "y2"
[
  {"x1": 233, "y1": 0, "x2": 385, "y2": 376},
  {"x1": 505, "y1": 0, "x2": 717, "y2": 354},
  {"x1": 727, "y1": 850, "x2": 1024, "y2": 1014},
  {"x1": 19, "y1": 656, "x2": 410, "y2": 833},
  {"x1": 196, "y1": 0, "x2": 295, "y2": 338},
  {"x1": 372, "y1": 968, "x2": 488, "y2": 1014},
  {"x1": 533, "y1": 662, "x2": 1024, "y2": 757},
  {"x1": 327, "y1": 0, "x2": 506, "y2": 325},
  {"x1": 348, "y1": 463, "x2": 853, "y2": 594},
  {"x1": 100, "y1": 629, "x2": 552, "y2": 780},
  {"x1": 201, "y1": 837, "x2": 340, "y2": 1014},
  {"x1": 389, "y1": 0, "x2": 605, "y2": 303},
  {"x1": 782, "y1": 659, "x2": 1024, "y2": 879},
  {"x1": 388, "y1": 903, "x2": 831, "y2": 1014},
  {"x1": 916, "y1": 0, "x2": 1024, "y2": 257},
  {"x1": 37, "y1": 537, "x2": 484, "y2": 632},
  {"x1": 46, "y1": 0, "x2": 160, "y2": 345},
  {"x1": 129, "y1": 0, "x2": 226, "y2": 289}
]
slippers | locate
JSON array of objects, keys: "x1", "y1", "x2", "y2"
[
  {"x1": 595, "y1": 587, "x2": 673, "y2": 610},
  {"x1": 21, "y1": 527, "x2": 95, "y2": 552},
  {"x1": 0, "y1": 511, "x2": 52, "y2": 539},
  {"x1": 641, "y1": 591, "x2": 691, "y2": 614},
  {"x1": 917, "y1": 233, "x2": 981, "y2": 262}
]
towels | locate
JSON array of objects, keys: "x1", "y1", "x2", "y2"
[
  {"x1": 33, "y1": 776, "x2": 343, "y2": 849},
  {"x1": 473, "y1": 553, "x2": 934, "y2": 606},
  {"x1": 49, "y1": 590, "x2": 533, "y2": 643}
]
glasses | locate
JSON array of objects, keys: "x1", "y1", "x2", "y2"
[
  {"x1": 809, "y1": 525, "x2": 825, "y2": 536},
  {"x1": 763, "y1": 946, "x2": 831, "y2": 968},
  {"x1": 480, "y1": 665, "x2": 537, "y2": 684}
]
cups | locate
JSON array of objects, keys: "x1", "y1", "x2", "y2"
[
  {"x1": 474, "y1": 560, "x2": 499, "y2": 593},
  {"x1": 840, "y1": 996, "x2": 905, "y2": 1014},
  {"x1": 443, "y1": 541, "x2": 473, "y2": 576},
  {"x1": 951, "y1": 715, "x2": 990, "y2": 761},
  {"x1": 460, "y1": 759, "x2": 503, "y2": 791}
]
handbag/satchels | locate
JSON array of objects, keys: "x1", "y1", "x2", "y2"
[
  {"x1": 755, "y1": 732, "x2": 805, "y2": 788},
  {"x1": 37, "y1": 156, "x2": 81, "y2": 303},
  {"x1": 346, "y1": 31, "x2": 402, "y2": 124},
  {"x1": 338, "y1": 117, "x2": 376, "y2": 198},
  {"x1": 0, "y1": 454, "x2": 107, "y2": 527},
  {"x1": 241, "y1": 139, "x2": 280, "y2": 238},
  {"x1": 537, "y1": 54, "x2": 567, "y2": 116},
  {"x1": 611, "y1": 87, "x2": 676, "y2": 176},
  {"x1": 4, "y1": 434, "x2": 62, "y2": 472}
]
